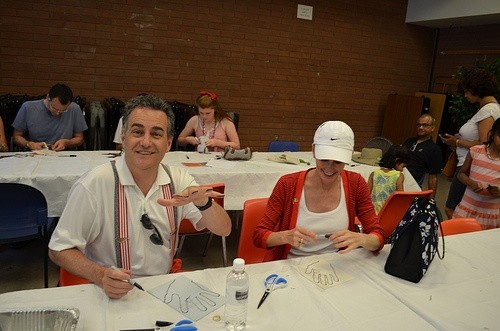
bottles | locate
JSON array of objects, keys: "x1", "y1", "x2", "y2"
[{"x1": 224, "y1": 258, "x2": 249, "y2": 331}]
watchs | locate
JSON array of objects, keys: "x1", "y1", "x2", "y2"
[{"x1": 25, "y1": 143, "x2": 28, "y2": 148}]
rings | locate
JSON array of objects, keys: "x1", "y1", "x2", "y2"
[{"x1": 300, "y1": 238, "x2": 303, "y2": 243}]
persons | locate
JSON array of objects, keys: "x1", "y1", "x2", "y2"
[
  {"x1": 177, "y1": 91, "x2": 240, "y2": 152},
  {"x1": 383, "y1": 113, "x2": 441, "y2": 194},
  {"x1": 365, "y1": 142, "x2": 409, "y2": 216},
  {"x1": 48, "y1": 95, "x2": 232, "y2": 299},
  {"x1": 451, "y1": 117, "x2": 500, "y2": 231},
  {"x1": 438, "y1": 70, "x2": 500, "y2": 221},
  {"x1": 253, "y1": 121, "x2": 384, "y2": 263},
  {"x1": 12, "y1": 83, "x2": 89, "y2": 151},
  {"x1": 0, "y1": 117, "x2": 8, "y2": 151}
]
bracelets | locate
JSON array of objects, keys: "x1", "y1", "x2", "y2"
[
  {"x1": 195, "y1": 197, "x2": 212, "y2": 211},
  {"x1": 456, "y1": 139, "x2": 459, "y2": 146}
]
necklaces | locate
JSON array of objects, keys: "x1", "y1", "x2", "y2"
[
  {"x1": 203, "y1": 118, "x2": 216, "y2": 139},
  {"x1": 487, "y1": 144, "x2": 500, "y2": 161}
]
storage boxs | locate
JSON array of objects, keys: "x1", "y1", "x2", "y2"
[{"x1": 0, "y1": 306, "x2": 79, "y2": 331}]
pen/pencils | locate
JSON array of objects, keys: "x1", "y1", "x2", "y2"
[
  {"x1": 350, "y1": 164, "x2": 361, "y2": 167},
  {"x1": 111, "y1": 265, "x2": 145, "y2": 292},
  {"x1": 185, "y1": 153, "x2": 189, "y2": 158},
  {"x1": 59, "y1": 154, "x2": 77, "y2": 157}
]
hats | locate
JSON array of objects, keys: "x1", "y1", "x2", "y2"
[
  {"x1": 351, "y1": 148, "x2": 383, "y2": 166},
  {"x1": 313, "y1": 120, "x2": 354, "y2": 165}
]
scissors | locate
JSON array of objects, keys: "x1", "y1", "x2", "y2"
[
  {"x1": 256, "y1": 273, "x2": 287, "y2": 310},
  {"x1": 120, "y1": 319, "x2": 198, "y2": 331}
]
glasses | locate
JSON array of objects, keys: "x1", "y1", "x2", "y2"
[
  {"x1": 140, "y1": 214, "x2": 163, "y2": 245},
  {"x1": 416, "y1": 122, "x2": 432, "y2": 127},
  {"x1": 49, "y1": 99, "x2": 68, "y2": 113}
]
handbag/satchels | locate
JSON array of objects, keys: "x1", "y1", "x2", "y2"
[
  {"x1": 385, "y1": 197, "x2": 445, "y2": 283},
  {"x1": 442, "y1": 151, "x2": 458, "y2": 176}
]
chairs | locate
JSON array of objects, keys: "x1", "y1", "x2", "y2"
[
  {"x1": 268, "y1": 142, "x2": 298, "y2": 152},
  {"x1": 366, "y1": 137, "x2": 392, "y2": 153},
  {"x1": 378, "y1": 189, "x2": 482, "y2": 244},
  {"x1": 0, "y1": 178, "x2": 273, "y2": 287}
]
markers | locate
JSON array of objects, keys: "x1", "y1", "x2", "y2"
[{"x1": 315, "y1": 234, "x2": 332, "y2": 239}]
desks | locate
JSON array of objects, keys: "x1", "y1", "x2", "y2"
[
  {"x1": 0, "y1": 228, "x2": 500, "y2": 331},
  {"x1": 0, "y1": 150, "x2": 421, "y2": 218}
]
